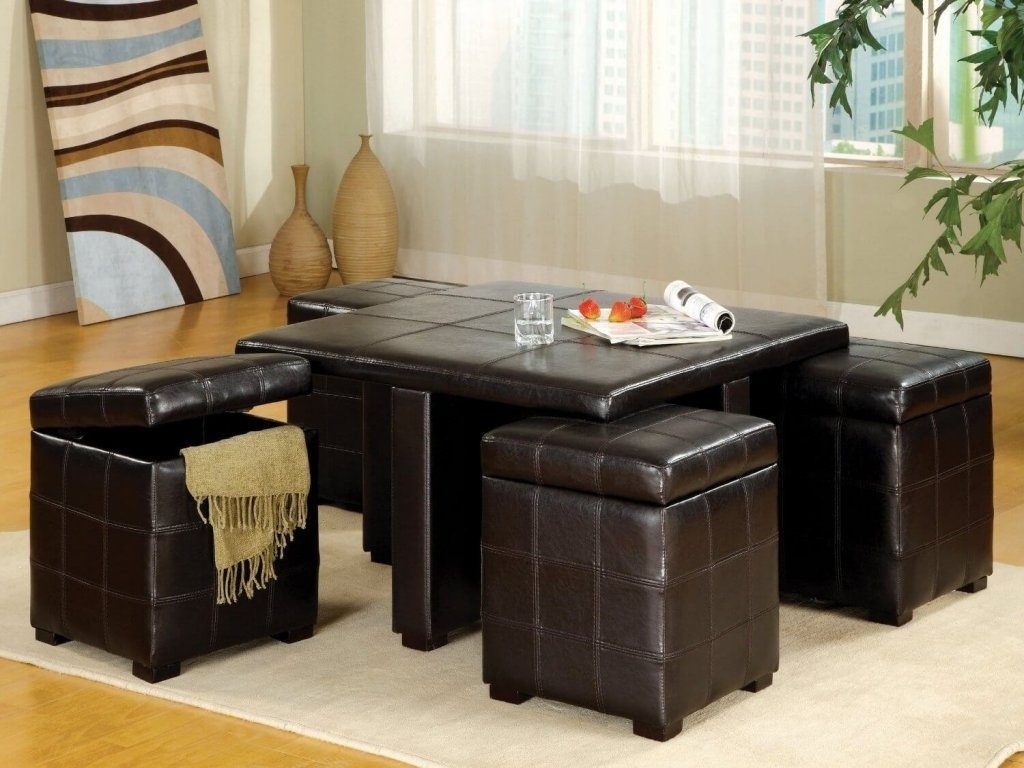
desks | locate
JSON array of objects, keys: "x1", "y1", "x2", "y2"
[{"x1": 234, "y1": 279, "x2": 852, "y2": 652}]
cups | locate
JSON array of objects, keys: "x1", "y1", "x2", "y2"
[{"x1": 513, "y1": 293, "x2": 554, "y2": 345}]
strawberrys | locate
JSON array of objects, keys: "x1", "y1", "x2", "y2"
[
  {"x1": 608, "y1": 301, "x2": 632, "y2": 322},
  {"x1": 579, "y1": 298, "x2": 601, "y2": 319},
  {"x1": 627, "y1": 297, "x2": 648, "y2": 319}
]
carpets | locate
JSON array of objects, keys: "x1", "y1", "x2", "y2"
[{"x1": 0, "y1": 504, "x2": 1024, "y2": 768}]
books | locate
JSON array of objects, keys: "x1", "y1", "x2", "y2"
[{"x1": 561, "y1": 280, "x2": 736, "y2": 347}]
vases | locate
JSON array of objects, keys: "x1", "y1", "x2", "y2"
[
  {"x1": 268, "y1": 165, "x2": 333, "y2": 303},
  {"x1": 331, "y1": 135, "x2": 400, "y2": 286}
]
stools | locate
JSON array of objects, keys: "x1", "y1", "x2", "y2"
[
  {"x1": 479, "y1": 404, "x2": 782, "y2": 743},
  {"x1": 751, "y1": 336, "x2": 994, "y2": 627},
  {"x1": 29, "y1": 351, "x2": 320, "y2": 685},
  {"x1": 285, "y1": 280, "x2": 466, "y2": 515}
]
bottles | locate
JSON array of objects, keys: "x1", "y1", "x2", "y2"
[
  {"x1": 269, "y1": 165, "x2": 332, "y2": 296},
  {"x1": 332, "y1": 134, "x2": 400, "y2": 285}
]
